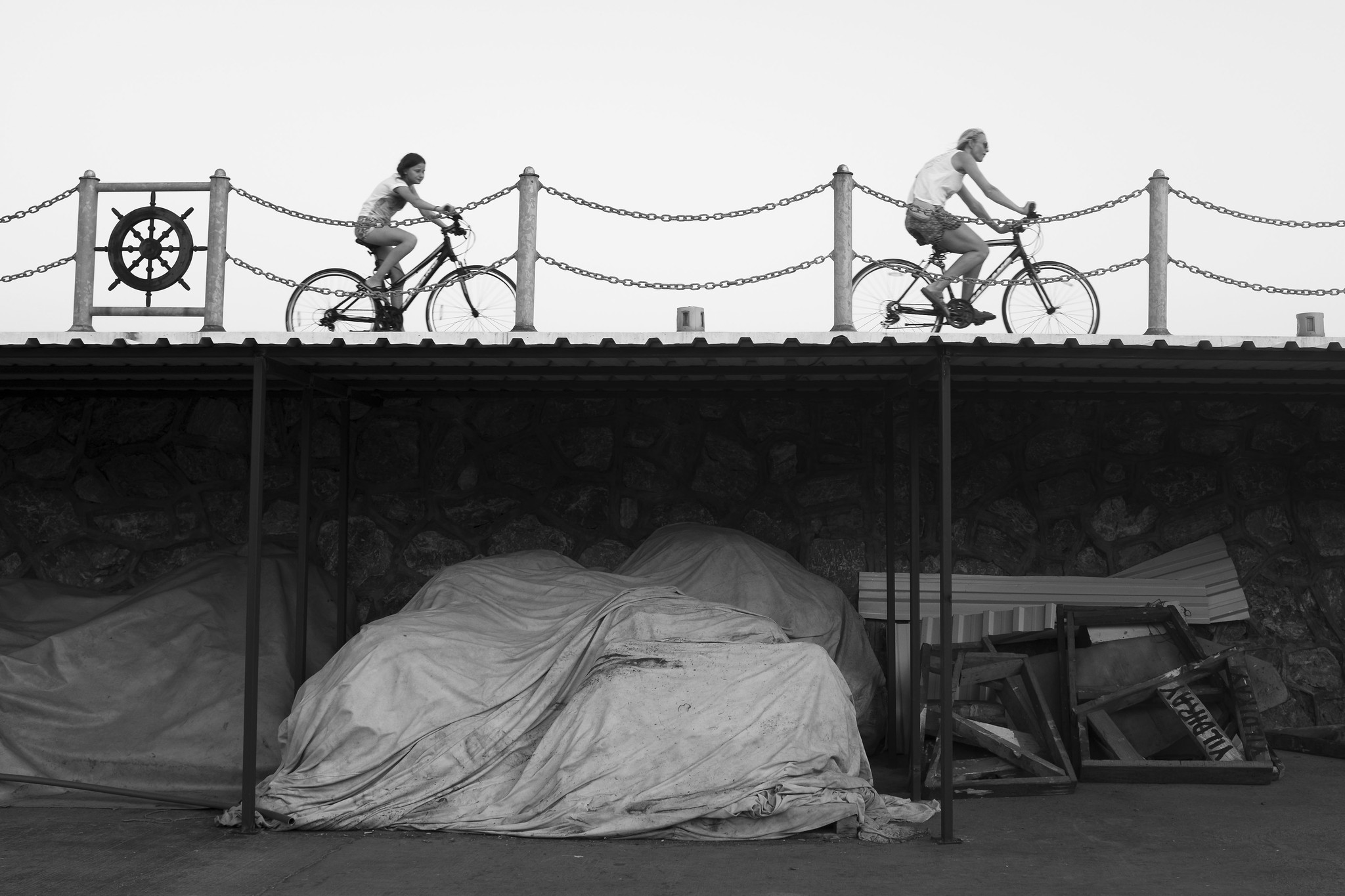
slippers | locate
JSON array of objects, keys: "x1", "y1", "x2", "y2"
[
  {"x1": 363, "y1": 283, "x2": 388, "y2": 293},
  {"x1": 973, "y1": 308, "x2": 996, "y2": 321},
  {"x1": 921, "y1": 287, "x2": 951, "y2": 319}
]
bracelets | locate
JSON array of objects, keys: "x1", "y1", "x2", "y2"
[{"x1": 433, "y1": 206, "x2": 439, "y2": 211}]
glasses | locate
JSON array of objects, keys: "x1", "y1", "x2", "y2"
[{"x1": 972, "y1": 139, "x2": 988, "y2": 148}]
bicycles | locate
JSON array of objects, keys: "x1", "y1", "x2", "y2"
[
  {"x1": 286, "y1": 205, "x2": 516, "y2": 332},
  {"x1": 852, "y1": 203, "x2": 1100, "y2": 334}
]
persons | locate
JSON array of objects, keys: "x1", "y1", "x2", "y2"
[
  {"x1": 905, "y1": 128, "x2": 1036, "y2": 323},
  {"x1": 354, "y1": 153, "x2": 457, "y2": 331}
]
